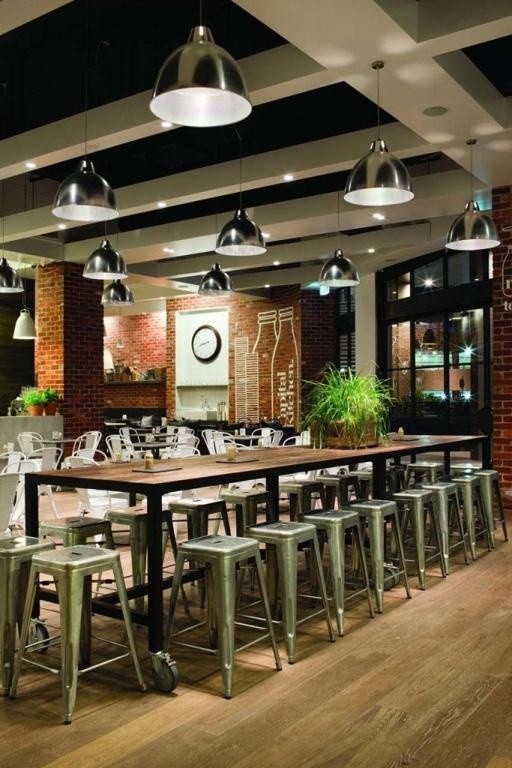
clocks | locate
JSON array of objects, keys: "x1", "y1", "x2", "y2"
[{"x1": 191, "y1": 324, "x2": 222, "y2": 364}]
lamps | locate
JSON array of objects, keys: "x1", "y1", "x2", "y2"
[
  {"x1": 98, "y1": 223, "x2": 135, "y2": 307},
  {"x1": 421, "y1": 319, "x2": 437, "y2": 346},
  {"x1": 444, "y1": 138, "x2": 502, "y2": 252},
  {"x1": 1, "y1": 215, "x2": 25, "y2": 295},
  {"x1": 81, "y1": 220, "x2": 129, "y2": 281},
  {"x1": 147, "y1": 1, "x2": 254, "y2": 128},
  {"x1": 13, "y1": 276, "x2": 37, "y2": 341},
  {"x1": 51, "y1": 83, "x2": 120, "y2": 222}
]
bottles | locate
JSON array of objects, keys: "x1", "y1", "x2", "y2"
[
  {"x1": 226, "y1": 442, "x2": 237, "y2": 460},
  {"x1": 40, "y1": 408, "x2": 46, "y2": 416},
  {"x1": 398, "y1": 426, "x2": 405, "y2": 438},
  {"x1": 54, "y1": 407, "x2": 60, "y2": 415},
  {"x1": 3, "y1": 444, "x2": 7, "y2": 453},
  {"x1": 59, "y1": 431, "x2": 63, "y2": 440},
  {"x1": 143, "y1": 448, "x2": 154, "y2": 469},
  {"x1": 235, "y1": 428, "x2": 238, "y2": 436}
]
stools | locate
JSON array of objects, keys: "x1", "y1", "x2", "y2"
[
  {"x1": 8, "y1": 544, "x2": 149, "y2": 725},
  {"x1": 1, "y1": 535, "x2": 58, "y2": 696}
]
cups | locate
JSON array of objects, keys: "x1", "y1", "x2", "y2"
[
  {"x1": 7, "y1": 441, "x2": 14, "y2": 452},
  {"x1": 240, "y1": 428, "x2": 246, "y2": 436},
  {"x1": 52, "y1": 430, "x2": 59, "y2": 439},
  {"x1": 144, "y1": 417, "x2": 167, "y2": 442}
]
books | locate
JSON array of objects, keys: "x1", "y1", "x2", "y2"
[
  {"x1": 132, "y1": 465, "x2": 181, "y2": 473},
  {"x1": 393, "y1": 435, "x2": 418, "y2": 442},
  {"x1": 217, "y1": 457, "x2": 259, "y2": 463}
]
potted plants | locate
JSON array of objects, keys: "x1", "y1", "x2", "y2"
[
  {"x1": 295, "y1": 360, "x2": 400, "y2": 450},
  {"x1": 22, "y1": 387, "x2": 48, "y2": 416},
  {"x1": 41, "y1": 388, "x2": 60, "y2": 417}
]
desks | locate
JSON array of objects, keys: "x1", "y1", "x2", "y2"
[{"x1": 22, "y1": 432, "x2": 492, "y2": 695}]
